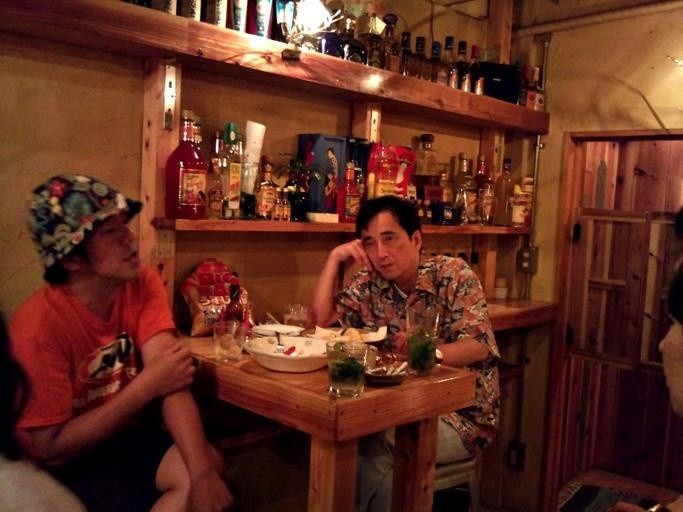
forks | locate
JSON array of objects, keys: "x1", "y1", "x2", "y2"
[{"x1": 273, "y1": 331, "x2": 285, "y2": 352}]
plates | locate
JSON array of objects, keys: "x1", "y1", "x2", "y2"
[
  {"x1": 250, "y1": 324, "x2": 304, "y2": 335},
  {"x1": 359, "y1": 348, "x2": 408, "y2": 384}
]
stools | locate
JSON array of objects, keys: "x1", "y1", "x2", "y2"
[{"x1": 432, "y1": 455, "x2": 484, "y2": 511}]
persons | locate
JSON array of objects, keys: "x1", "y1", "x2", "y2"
[
  {"x1": 606, "y1": 256, "x2": 682, "y2": 511},
  {"x1": 312, "y1": 195, "x2": 503, "y2": 511},
  {"x1": 6, "y1": 170, "x2": 236, "y2": 511},
  {"x1": 0, "y1": 306, "x2": 86, "y2": 511}
]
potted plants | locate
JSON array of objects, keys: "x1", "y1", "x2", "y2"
[{"x1": 270, "y1": 152, "x2": 330, "y2": 222}]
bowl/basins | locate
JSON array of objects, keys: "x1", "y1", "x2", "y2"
[
  {"x1": 301, "y1": 327, "x2": 391, "y2": 350},
  {"x1": 243, "y1": 335, "x2": 326, "y2": 374}
]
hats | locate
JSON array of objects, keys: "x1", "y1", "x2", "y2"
[{"x1": 24, "y1": 172, "x2": 142, "y2": 285}]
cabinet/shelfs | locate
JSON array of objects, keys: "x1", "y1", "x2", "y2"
[{"x1": 139, "y1": 5, "x2": 557, "y2": 335}]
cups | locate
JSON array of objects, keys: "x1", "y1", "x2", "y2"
[
  {"x1": 405, "y1": 306, "x2": 440, "y2": 375},
  {"x1": 494, "y1": 278, "x2": 508, "y2": 299},
  {"x1": 326, "y1": 341, "x2": 368, "y2": 396},
  {"x1": 212, "y1": 320, "x2": 241, "y2": 362},
  {"x1": 232, "y1": 302, "x2": 254, "y2": 355},
  {"x1": 350, "y1": 344, "x2": 378, "y2": 369},
  {"x1": 282, "y1": 304, "x2": 311, "y2": 327}
]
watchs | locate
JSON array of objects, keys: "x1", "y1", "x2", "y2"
[{"x1": 435, "y1": 344, "x2": 443, "y2": 362}]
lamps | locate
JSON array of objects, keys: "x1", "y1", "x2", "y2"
[{"x1": 281, "y1": 0, "x2": 345, "y2": 61}]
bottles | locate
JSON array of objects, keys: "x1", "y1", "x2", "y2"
[
  {"x1": 148, "y1": 0, "x2": 486, "y2": 98},
  {"x1": 225, "y1": 283, "x2": 242, "y2": 333},
  {"x1": 524, "y1": 67, "x2": 545, "y2": 111},
  {"x1": 166, "y1": 112, "x2": 292, "y2": 220},
  {"x1": 413, "y1": 134, "x2": 531, "y2": 226},
  {"x1": 336, "y1": 160, "x2": 360, "y2": 223},
  {"x1": 429, "y1": 249, "x2": 482, "y2": 286}
]
